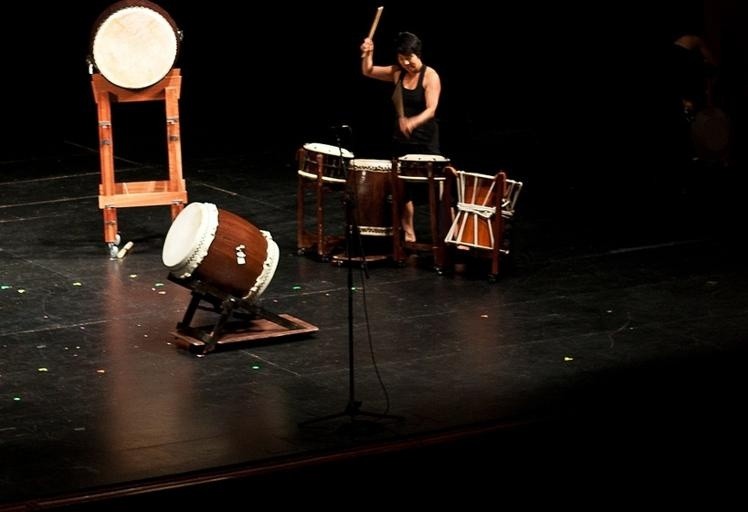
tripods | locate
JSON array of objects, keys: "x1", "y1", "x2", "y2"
[{"x1": 297, "y1": 140, "x2": 407, "y2": 428}]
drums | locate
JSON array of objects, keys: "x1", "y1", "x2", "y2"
[
  {"x1": 296, "y1": 143, "x2": 354, "y2": 182},
  {"x1": 89, "y1": 0, "x2": 186, "y2": 91},
  {"x1": 162, "y1": 201, "x2": 279, "y2": 303},
  {"x1": 341, "y1": 159, "x2": 403, "y2": 238},
  {"x1": 397, "y1": 153, "x2": 450, "y2": 181},
  {"x1": 444, "y1": 171, "x2": 523, "y2": 255}
]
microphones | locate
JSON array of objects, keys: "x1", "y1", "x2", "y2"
[{"x1": 331, "y1": 124, "x2": 353, "y2": 137}]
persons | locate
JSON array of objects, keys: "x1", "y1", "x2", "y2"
[{"x1": 360, "y1": 32, "x2": 470, "y2": 250}]
